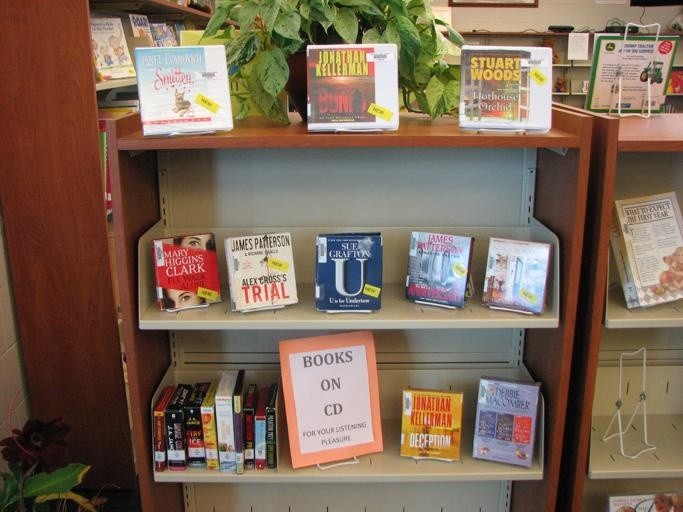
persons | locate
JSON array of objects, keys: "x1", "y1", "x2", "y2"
[
  {"x1": 651, "y1": 495, "x2": 683, "y2": 512},
  {"x1": 161, "y1": 288, "x2": 202, "y2": 309},
  {"x1": 174, "y1": 235, "x2": 214, "y2": 254}
]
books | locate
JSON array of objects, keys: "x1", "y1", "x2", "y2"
[{"x1": 612, "y1": 193, "x2": 683, "y2": 308}]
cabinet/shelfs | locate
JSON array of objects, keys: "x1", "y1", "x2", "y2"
[
  {"x1": 439, "y1": 31, "x2": 682, "y2": 112},
  {"x1": 106, "y1": 106, "x2": 594, "y2": 512},
  {"x1": 0, "y1": 1, "x2": 244, "y2": 512},
  {"x1": 569, "y1": 100, "x2": 683, "y2": 512}
]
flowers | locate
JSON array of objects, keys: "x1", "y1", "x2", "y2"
[{"x1": 0, "y1": 417, "x2": 118, "y2": 512}]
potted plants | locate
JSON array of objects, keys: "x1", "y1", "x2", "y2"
[{"x1": 204, "y1": 0, "x2": 462, "y2": 125}]
